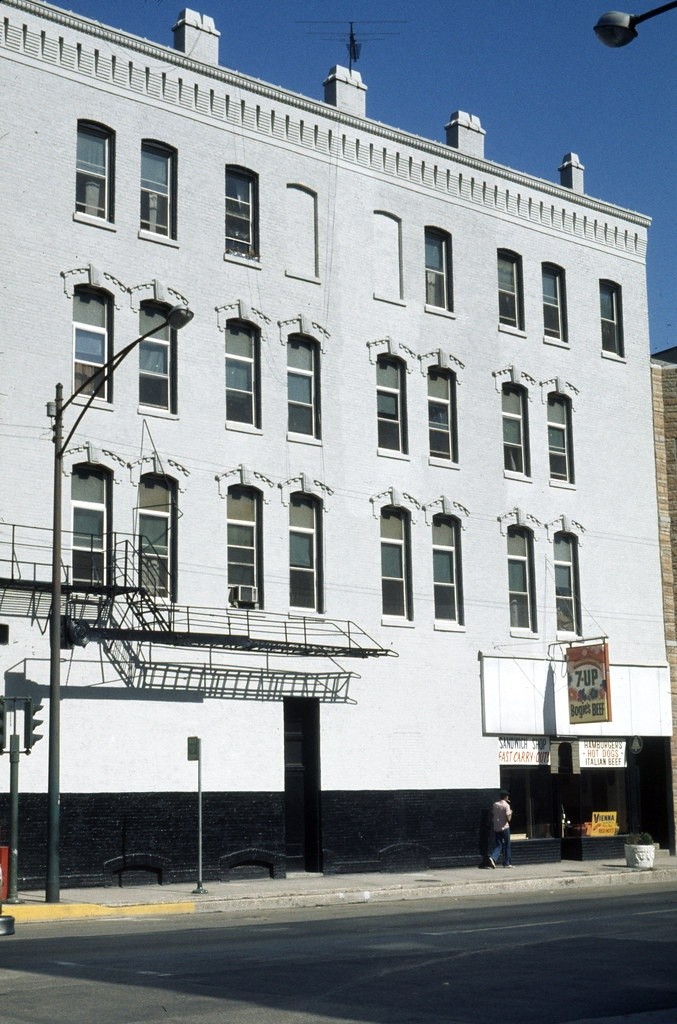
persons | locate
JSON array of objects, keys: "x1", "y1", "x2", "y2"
[{"x1": 489, "y1": 791, "x2": 515, "y2": 869}]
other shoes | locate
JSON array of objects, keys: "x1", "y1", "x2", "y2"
[
  {"x1": 503, "y1": 864, "x2": 515, "y2": 868},
  {"x1": 489, "y1": 857, "x2": 496, "y2": 870}
]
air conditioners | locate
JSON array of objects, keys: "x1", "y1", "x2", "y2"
[{"x1": 232, "y1": 585, "x2": 258, "y2": 603}]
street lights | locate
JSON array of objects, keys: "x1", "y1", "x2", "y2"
[{"x1": 43, "y1": 306, "x2": 194, "y2": 905}]
